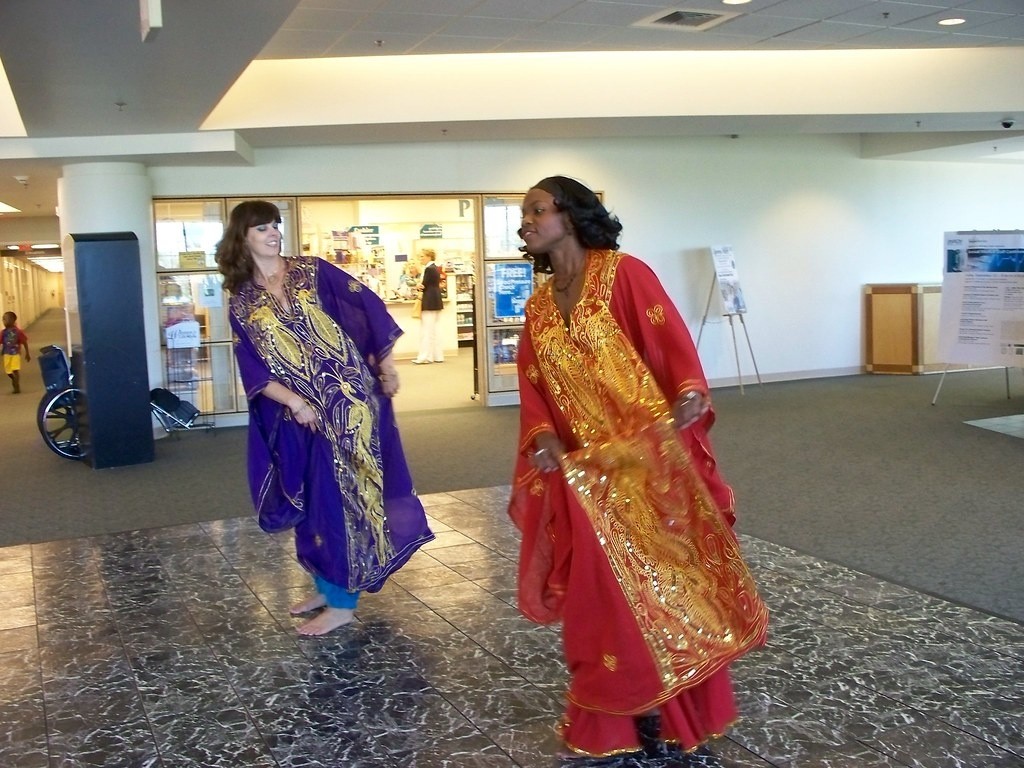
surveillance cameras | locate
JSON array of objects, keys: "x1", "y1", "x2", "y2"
[{"x1": 1002, "y1": 120, "x2": 1015, "y2": 128}]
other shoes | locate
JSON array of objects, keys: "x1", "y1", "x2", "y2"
[
  {"x1": 411, "y1": 359, "x2": 430, "y2": 364},
  {"x1": 434, "y1": 361, "x2": 443, "y2": 363}
]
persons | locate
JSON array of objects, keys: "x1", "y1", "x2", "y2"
[
  {"x1": 504, "y1": 174, "x2": 771, "y2": 760},
  {"x1": 0, "y1": 311, "x2": 31, "y2": 394},
  {"x1": 400, "y1": 246, "x2": 446, "y2": 364},
  {"x1": 216, "y1": 201, "x2": 440, "y2": 638}
]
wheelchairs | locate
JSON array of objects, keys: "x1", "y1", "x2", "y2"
[{"x1": 36, "y1": 345, "x2": 217, "y2": 462}]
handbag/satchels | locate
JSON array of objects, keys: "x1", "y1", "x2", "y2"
[{"x1": 411, "y1": 289, "x2": 422, "y2": 319}]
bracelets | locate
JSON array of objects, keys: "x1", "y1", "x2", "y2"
[
  {"x1": 381, "y1": 373, "x2": 398, "y2": 382},
  {"x1": 293, "y1": 403, "x2": 309, "y2": 417}
]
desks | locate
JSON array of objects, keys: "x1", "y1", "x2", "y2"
[{"x1": 867, "y1": 283, "x2": 1013, "y2": 375}]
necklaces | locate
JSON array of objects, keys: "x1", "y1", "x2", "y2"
[
  {"x1": 251, "y1": 259, "x2": 284, "y2": 284},
  {"x1": 553, "y1": 251, "x2": 587, "y2": 297}
]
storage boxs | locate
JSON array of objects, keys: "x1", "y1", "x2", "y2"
[
  {"x1": 419, "y1": 223, "x2": 443, "y2": 238},
  {"x1": 157, "y1": 280, "x2": 201, "y2": 415},
  {"x1": 333, "y1": 226, "x2": 380, "y2": 249}
]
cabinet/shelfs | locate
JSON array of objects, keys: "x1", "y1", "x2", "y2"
[
  {"x1": 332, "y1": 236, "x2": 387, "y2": 299},
  {"x1": 456, "y1": 273, "x2": 474, "y2": 341}
]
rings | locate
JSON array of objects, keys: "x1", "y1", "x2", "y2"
[
  {"x1": 536, "y1": 449, "x2": 548, "y2": 457},
  {"x1": 679, "y1": 390, "x2": 696, "y2": 405}
]
